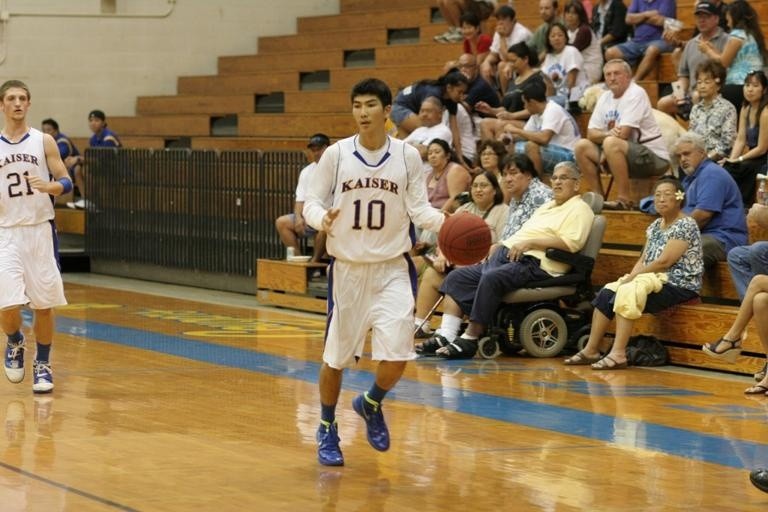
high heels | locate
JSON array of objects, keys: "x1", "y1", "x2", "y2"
[
  {"x1": 755, "y1": 361, "x2": 768, "y2": 382},
  {"x1": 702, "y1": 336, "x2": 744, "y2": 364}
]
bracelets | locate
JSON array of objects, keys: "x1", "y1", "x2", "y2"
[
  {"x1": 55, "y1": 177, "x2": 71, "y2": 197},
  {"x1": 75, "y1": 154, "x2": 82, "y2": 163}
]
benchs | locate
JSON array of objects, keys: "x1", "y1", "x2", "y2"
[{"x1": 42, "y1": 2, "x2": 767, "y2": 373}]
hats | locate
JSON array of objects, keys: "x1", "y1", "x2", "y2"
[
  {"x1": 307, "y1": 132, "x2": 330, "y2": 146},
  {"x1": 694, "y1": 1, "x2": 718, "y2": 15}
]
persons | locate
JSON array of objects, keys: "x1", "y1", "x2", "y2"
[
  {"x1": 295, "y1": 72, "x2": 471, "y2": 473},
  {"x1": 0, "y1": 77, "x2": 73, "y2": 398},
  {"x1": 64, "y1": 107, "x2": 122, "y2": 210},
  {"x1": 39, "y1": 116, "x2": 80, "y2": 186},
  {"x1": 276, "y1": 2, "x2": 768, "y2": 395}
]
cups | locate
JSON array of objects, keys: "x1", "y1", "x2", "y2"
[
  {"x1": 287, "y1": 247, "x2": 293, "y2": 261},
  {"x1": 671, "y1": 81, "x2": 687, "y2": 105}
]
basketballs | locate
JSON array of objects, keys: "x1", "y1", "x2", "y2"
[{"x1": 439, "y1": 212, "x2": 491, "y2": 265}]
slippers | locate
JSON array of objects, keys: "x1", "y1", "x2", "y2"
[
  {"x1": 744, "y1": 384, "x2": 768, "y2": 394},
  {"x1": 591, "y1": 356, "x2": 627, "y2": 370},
  {"x1": 564, "y1": 350, "x2": 601, "y2": 364}
]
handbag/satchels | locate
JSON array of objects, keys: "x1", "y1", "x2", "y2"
[{"x1": 626, "y1": 334, "x2": 667, "y2": 367}]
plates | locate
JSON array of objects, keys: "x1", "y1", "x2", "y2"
[{"x1": 288, "y1": 256, "x2": 312, "y2": 263}]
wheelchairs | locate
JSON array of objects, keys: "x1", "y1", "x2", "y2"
[{"x1": 477, "y1": 189, "x2": 608, "y2": 359}]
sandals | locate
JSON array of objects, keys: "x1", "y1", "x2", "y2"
[
  {"x1": 604, "y1": 197, "x2": 634, "y2": 211},
  {"x1": 437, "y1": 336, "x2": 483, "y2": 359},
  {"x1": 415, "y1": 334, "x2": 450, "y2": 355}
]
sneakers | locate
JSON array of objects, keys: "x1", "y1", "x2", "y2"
[
  {"x1": 316, "y1": 419, "x2": 344, "y2": 466},
  {"x1": 750, "y1": 468, "x2": 768, "y2": 493},
  {"x1": 3, "y1": 329, "x2": 26, "y2": 382},
  {"x1": 352, "y1": 391, "x2": 389, "y2": 451},
  {"x1": 32, "y1": 352, "x2": 54, "y2": 393},
  {"x1": 67, "y1": 199, "x2": 95, "y2": 210}
]
